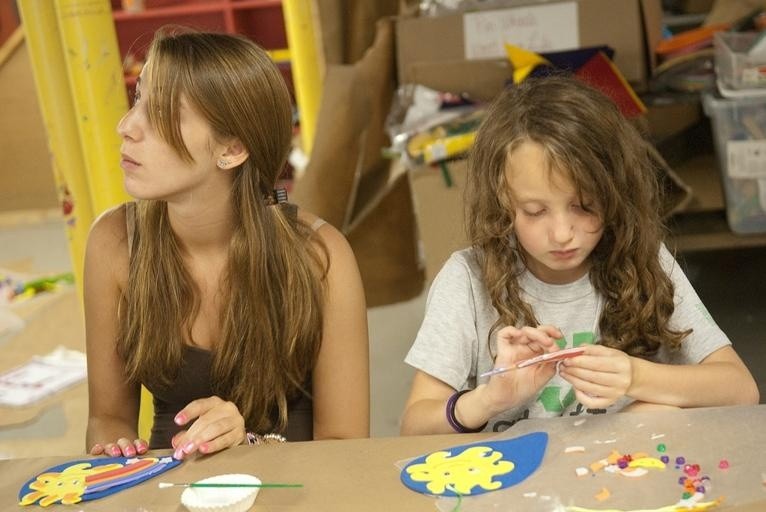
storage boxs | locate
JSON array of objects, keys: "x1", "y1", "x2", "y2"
[
  {"x1": 711, "y1": 32, "x2": 766, "y2": 97},
  {"x1": 397, "y1": 3, "x2": 644, "y2": 106},
  {"x1": 407, "y1": 156, "x2": 477, "y2": 281},
  {"x1": 698, "y1": 91, "x2": 763, "y2": 235}
]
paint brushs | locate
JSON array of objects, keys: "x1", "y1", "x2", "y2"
[{"x1": 158, "y1": 482, "x2": 304, "y2": 489}]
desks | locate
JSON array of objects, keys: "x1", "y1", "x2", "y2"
[{"x1": 0, "y1": 404, "x2": 766, "y2": 512}]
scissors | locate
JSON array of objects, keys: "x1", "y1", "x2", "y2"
[{"x1": 479, "y1": 347, "x2": 585, "y2": 377}]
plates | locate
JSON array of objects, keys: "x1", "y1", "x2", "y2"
[{"x1": 180, "y1": 474, "x2": 262, "y2": 512}]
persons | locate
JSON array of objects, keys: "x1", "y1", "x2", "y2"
[
  {"x1": 399, "y1": 73, "x2": 761, "y2": 438},
  {"x1": 83, "y1": 21, "x2": 371, "y2": 460}
]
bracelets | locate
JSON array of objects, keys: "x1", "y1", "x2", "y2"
[
  {"x1": 242, "y1": 431, "x2": 288, "y2": 449},
  {"x1": 447, "y1": 388, "x2": 490, "y2": 435}
]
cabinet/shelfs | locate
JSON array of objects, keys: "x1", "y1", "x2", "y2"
[{"x1": 108, "y1": 0, "x2": 327, "y2": 196}]
